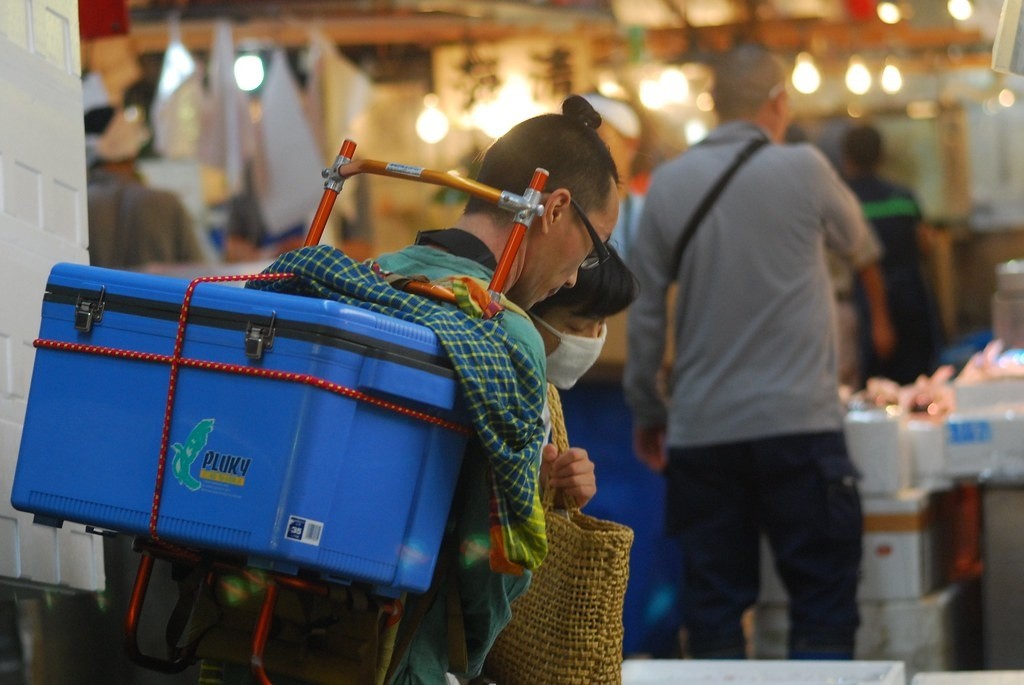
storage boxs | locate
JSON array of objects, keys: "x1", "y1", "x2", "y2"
[
  {"x1": 10, "y1": 259, "x2": 470, "y2": 601},
  {"x1": 617, "y1": 379, "x2": 1024, "y2": 684}
]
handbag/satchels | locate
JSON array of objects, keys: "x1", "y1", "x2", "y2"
[{"x1": 480, "y1": 383, "x2": 634, "y2": 685}]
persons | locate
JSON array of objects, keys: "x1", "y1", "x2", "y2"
[
  {"x1": 578, "y1": 95, "x2": 648, "y2": 265},
  {"x1": 191, "y1": 94, "x2": 621, "y2": 685},
  {"x1": 86, "y1": 108, "x2": 207, "y2": 271},
  {"x1": 626, "y1": 38, "x2": 885, "y2": 662},
  {"x1": 834, "y1": 121, "x2": 940, "y2": 391},
  {"x1": 462, "y1": 238, "x2": 642, "y2": 685}
]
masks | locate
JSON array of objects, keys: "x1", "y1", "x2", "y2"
[{"x1": 528, "y1": 310, "x2": 608, "y2": 390}]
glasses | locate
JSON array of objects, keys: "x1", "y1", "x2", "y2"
[{"x1": 571, "y1": 198, "x2": 610, "y2": 269}]
